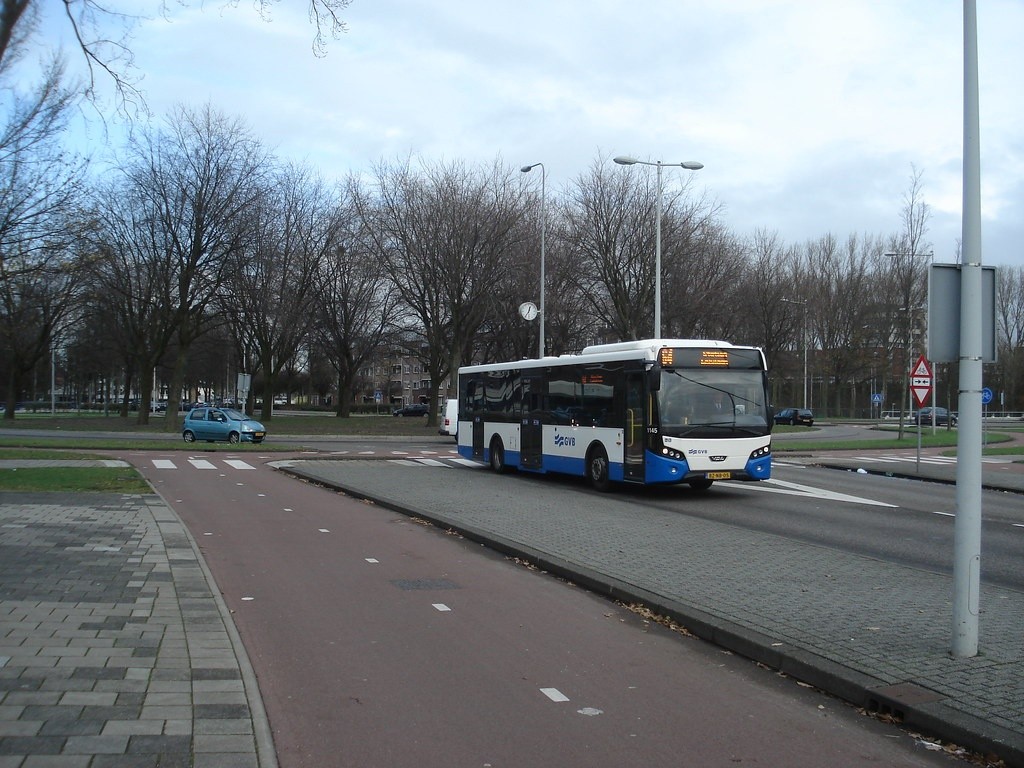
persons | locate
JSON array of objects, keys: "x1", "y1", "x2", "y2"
[{"x1": 709, "y1": 390, "x2": 728, "y2": 415}]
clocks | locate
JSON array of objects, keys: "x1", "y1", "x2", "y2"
[{"x1": 518, "y1": 302, "x2": 538, "y2": 321}]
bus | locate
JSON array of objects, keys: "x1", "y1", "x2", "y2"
[{"x1": 457, "y1": 340, "x2": 774, "y2": 492}]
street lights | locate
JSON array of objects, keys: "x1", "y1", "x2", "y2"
[
  {"x1": 520, "y1": 163, "x2": 545, "y2": 358},
  {"x1": 884, "y1": 251, "x2": 936, "y2": 436},
  {"x1": 613, "y1": 156, "x2": 705, "y2": 339},
  {"x1": 780, "y1": 298, "x2": 807, "y2": 410}
]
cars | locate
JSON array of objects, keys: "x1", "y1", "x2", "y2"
[
  {"x1": 393, "y1": 404, "x2": 430, "y2": 417},
  {"x1": 774, "y1": 408, "x2": 815, "y2": 427},
  {"x1": 223, "y1": 398, "x2": 296, "y2": 404},
  {"x1": 115, "y1": 401, "x2": 218, "y2": 412},
  {"x1": 915, "y1": 407, "x2": 958, "y2": 427},
  {"x1": 182, "y1": 407, "x2": 267, "y2": 444}
]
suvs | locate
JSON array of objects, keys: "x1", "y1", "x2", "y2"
[{"x1": 438, "y1": 399, "x2": 458, "y2": 441}]
